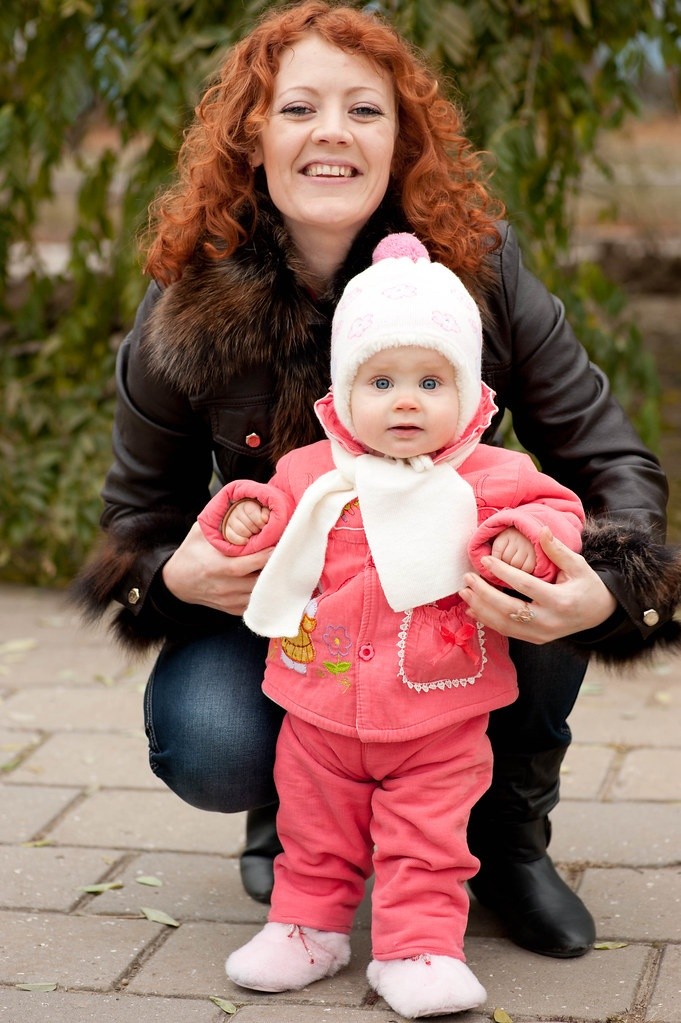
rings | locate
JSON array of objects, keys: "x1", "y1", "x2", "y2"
[{"x1": 510, "y1": 602, "x2": 536, "y2": 624}]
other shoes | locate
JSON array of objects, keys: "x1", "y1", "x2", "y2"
[
  {"x1": 366, "y1": 953, "x2": 488, "y2": 1019},
  {"x1": 226, "y1": 921, "x2": 351, "y2": 992},
  {"x1": 466, "y1": 851, "x2": 596, "y2": 959},
  {"x1": 238, "y1": 801, "x2": 284, "y2": 905}
]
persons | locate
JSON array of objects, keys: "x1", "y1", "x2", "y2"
[
  {"x1": 196, "y1": 233, "x2": 589, "y2": 1021},
  {"x1": 74, "y1": 3, "x2": 678, "y2": 960}
]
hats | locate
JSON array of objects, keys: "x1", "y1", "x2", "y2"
[{"x1": 330, "y1": 232, "x2": 481, "y2": 457}]
